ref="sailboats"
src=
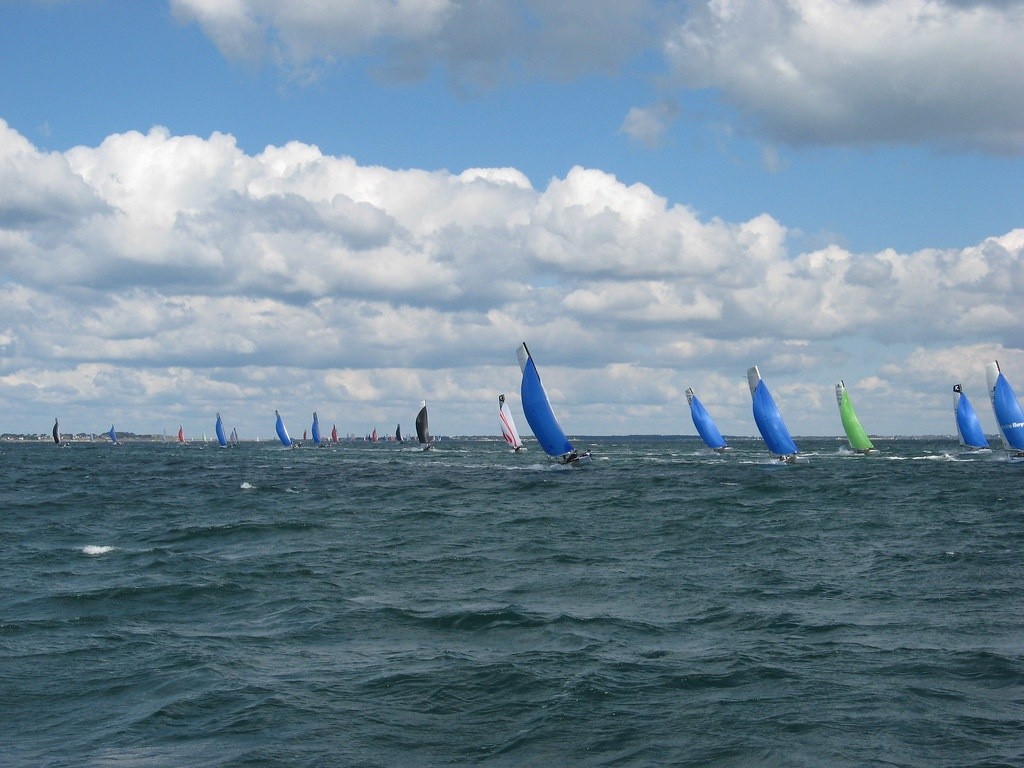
[685,388,735,453]
[108,424,125,446]
[52,417,69,447]
[985,360,1024,459]
[276,409,302,451]
[215,413,233,449]
[498,394,528,453]
[952,383,992,455]
[515,342,592,471]
[364,424,412,444]
[415,400,431,450]
[835,379,881,457]
[747,365,810,463]
[332,424,357,444]
[312,411,331,449]
